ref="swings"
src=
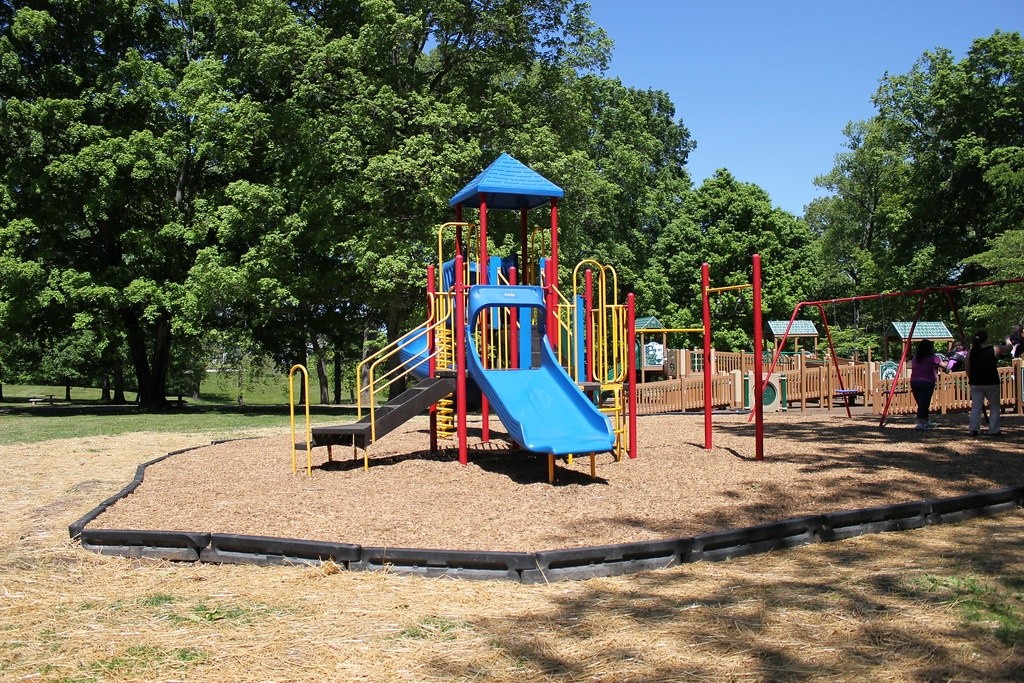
[944,286,1003,373]
[833,299,864,395]
[880,294,910,395]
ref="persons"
[910,339,947,430]
[945,325,1024,372]
[965,331,1013,437]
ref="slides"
[393,325,432,380]
[465,320,616,460]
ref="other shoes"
[970,430,978,436]
[924,420,937,428]
[990,433,1003,436]
[916,424,932,432]
